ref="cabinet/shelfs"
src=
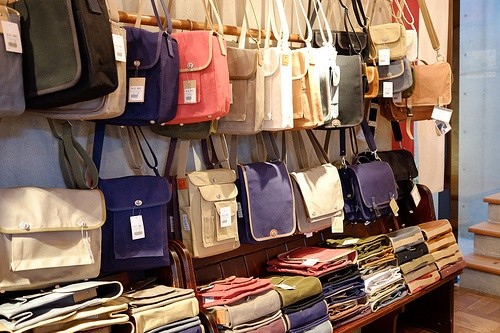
[166,181,469,333]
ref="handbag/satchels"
[335,121,399,226]
[153,0,455,128]
[232,132,297,245]
[0,120,107,292]
[26,0,127,123]
[0,218,468,333]
[350,116,420,205]
[0,0,26,117]
[91,124,173,277]
[175,133,241,259]
[85,0,180,127]
[6,0,119,109]
[285,129,347,237]
